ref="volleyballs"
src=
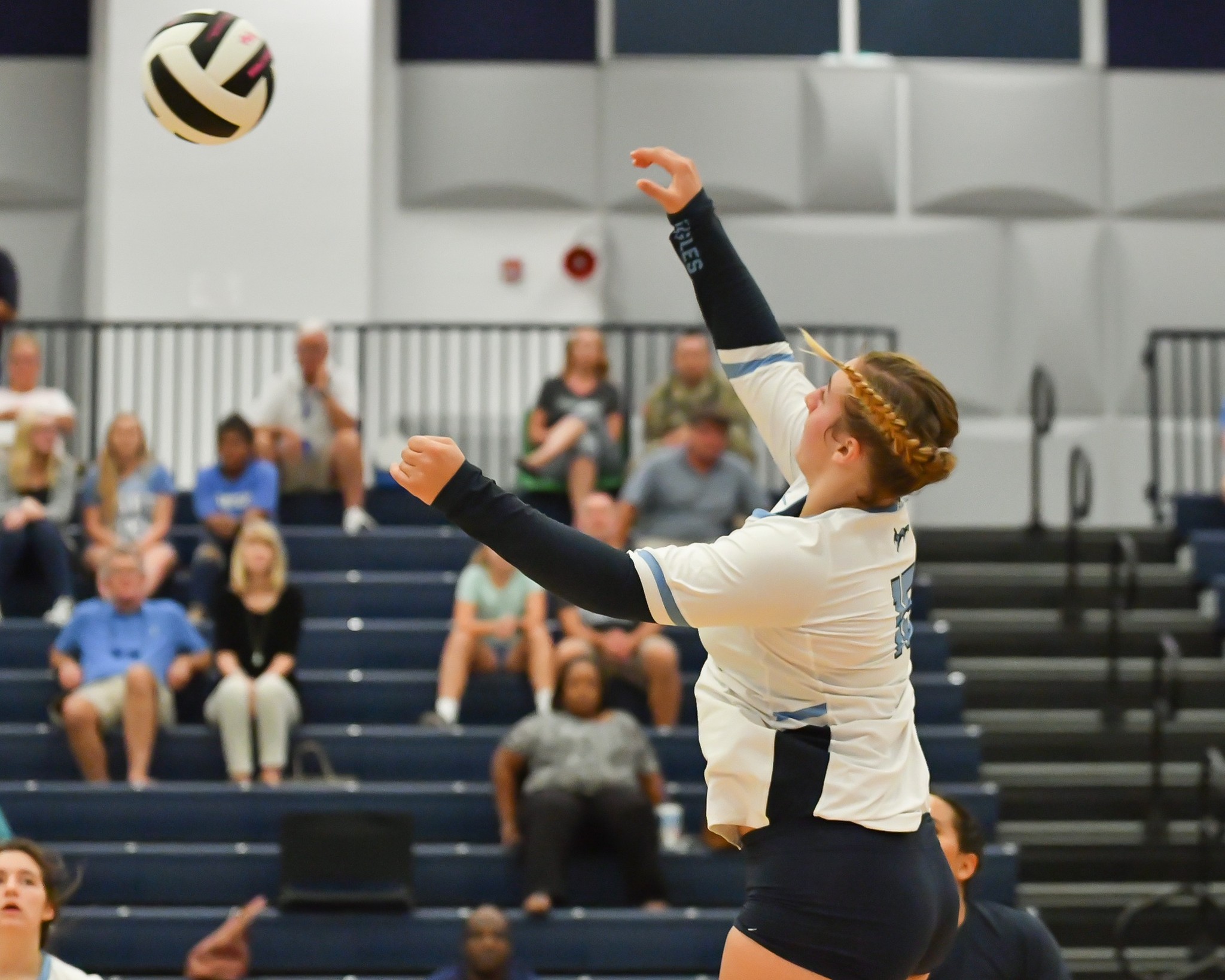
[139,10,275,143]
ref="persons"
[416,324,766,729]
[242,320,379,539]
[0,840,269,980]
[389,143,960,980]
[0,338,304,788]
[924,789,1065,980]
[485,659,674,917]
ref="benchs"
[0,490,1027,980]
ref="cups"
[657,804,685,848]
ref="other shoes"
[418,709,464,735]
[42,598,73,628]
